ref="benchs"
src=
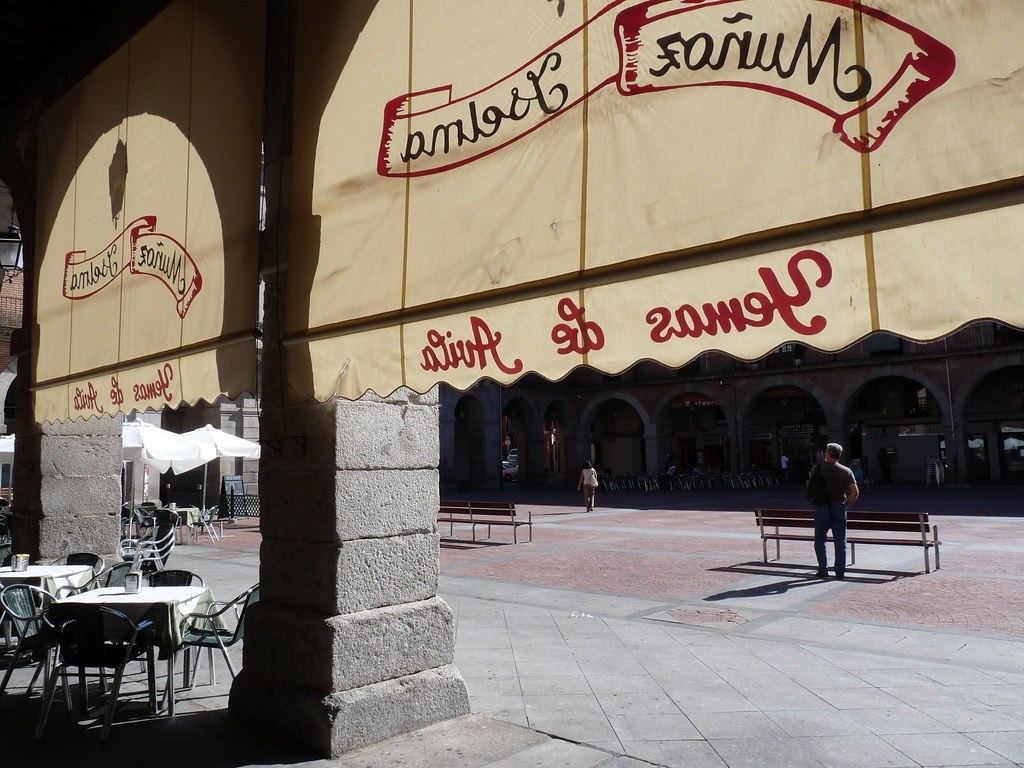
[754,507,941,574]
[438,500,533,545]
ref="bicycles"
[596,469,780,492]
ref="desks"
[32,586,229,719]
[154,508,199,545]
[0,565,100,649]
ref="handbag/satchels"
[591,469,598,487]
[807,464,827,506]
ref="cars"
[507,449,518,463]
[502,461,518,482]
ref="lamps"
[0,202,24,284]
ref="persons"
[664,465,676,491]
[806,443,864,581]
[577,461,599,513]
[781,452,790,484]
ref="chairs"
[597,469,780,492]
[0,501,259,742]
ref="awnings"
[0,0,1023,428]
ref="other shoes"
[836,573,844,579]
[591,509,593,511]
[816,571,828,576]
[587,509,590,512]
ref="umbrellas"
[121,419,261,521]
